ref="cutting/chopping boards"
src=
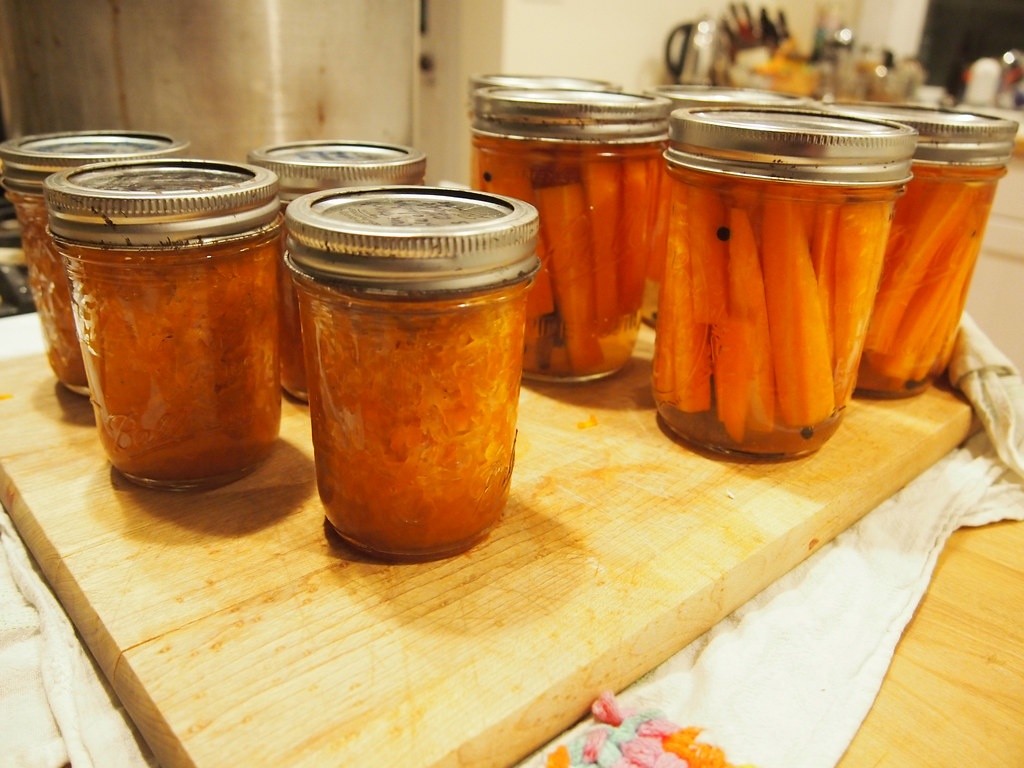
[1,311,984,768]
